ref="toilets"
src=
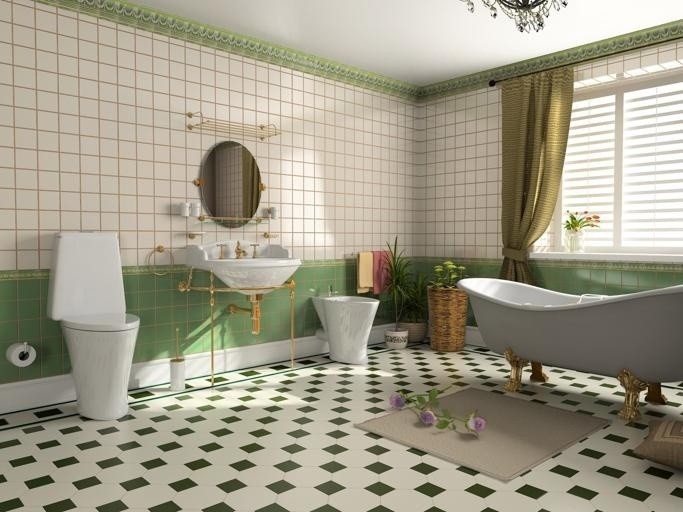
[46,232,142,422]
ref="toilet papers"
[5,342,37,367]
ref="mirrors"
[192,140,267,229]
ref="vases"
[569,230,583,253]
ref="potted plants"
[376,234,469,353]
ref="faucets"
[328,284,338,297]
[235,239,248,258]
[309,286,319,296]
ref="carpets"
[352,385,613,483]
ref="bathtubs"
[454,278,682,385]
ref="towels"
[354,250,391,295]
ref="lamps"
[459,0,569,35]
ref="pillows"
[633,418,683,469]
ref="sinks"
[312,294,381,363]
[209,254,303,294]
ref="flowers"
[560,208,601,235]
[386,385,486,439]
